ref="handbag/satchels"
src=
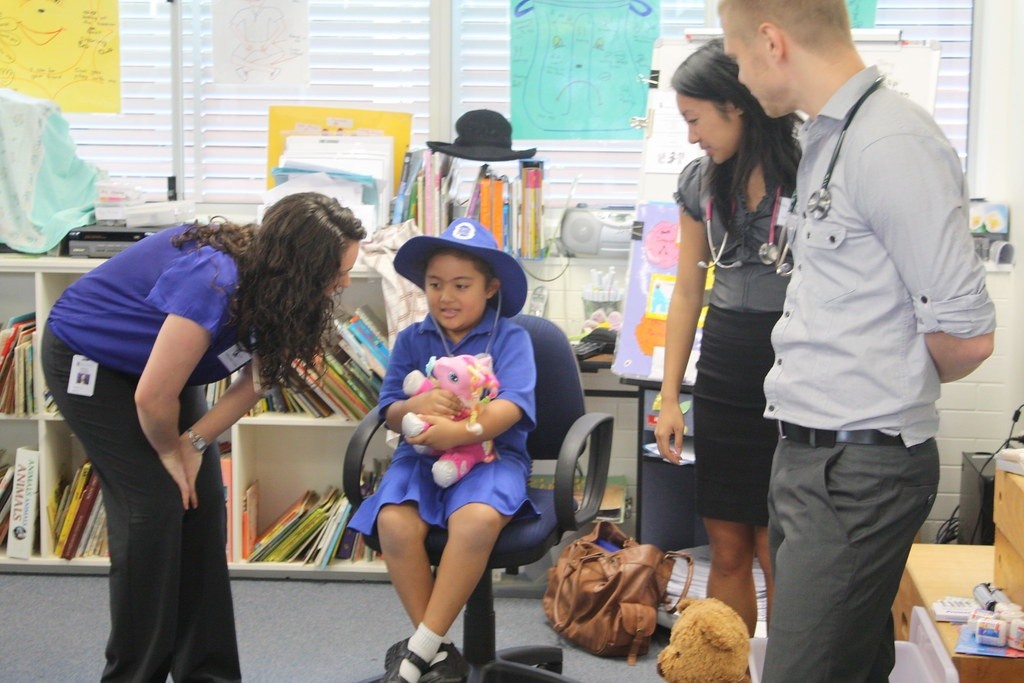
[544,521,693,665]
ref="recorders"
[560,203,635,259]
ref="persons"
[346,217,541,683]
[41,192,367,683]
[717,0,996,683]
[652,39,807,638]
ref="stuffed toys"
[654,597,752,683]
[401,352,500,487]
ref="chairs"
[340,315,616,683]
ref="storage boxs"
[889,466,1024,682]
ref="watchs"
[185,428,207,454]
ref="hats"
[426,110,535,160]
[394,218,526,317]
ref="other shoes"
[418,642,467,683]
[380,637,430,683]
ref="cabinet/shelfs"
[0,255,437,584]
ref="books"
[393,146,546,261]
[0,309,43,563]
[43,362,234,566]
[241,305,390,573]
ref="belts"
[776,419,903,448]
